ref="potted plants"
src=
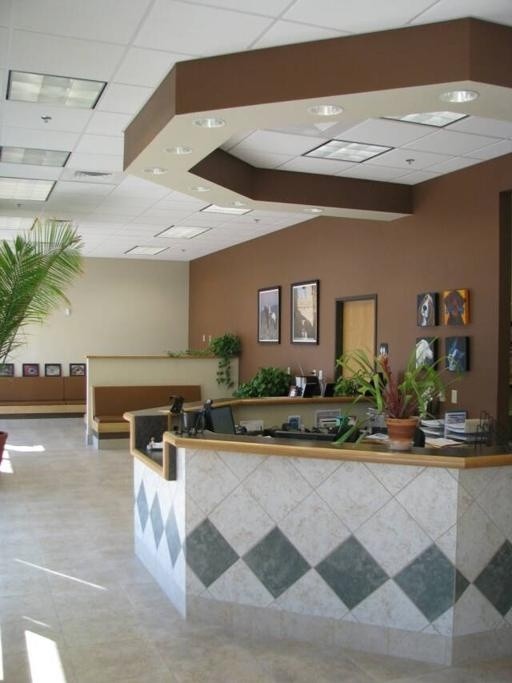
[0,216,86,462]
[332,337,461,452]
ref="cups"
[288,422,298,432]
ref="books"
[417,412,491,450]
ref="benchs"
[91,384,203,450]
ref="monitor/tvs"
[210,406,235,435]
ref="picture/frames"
[257,280,320,343]
[0,363,86,377]
